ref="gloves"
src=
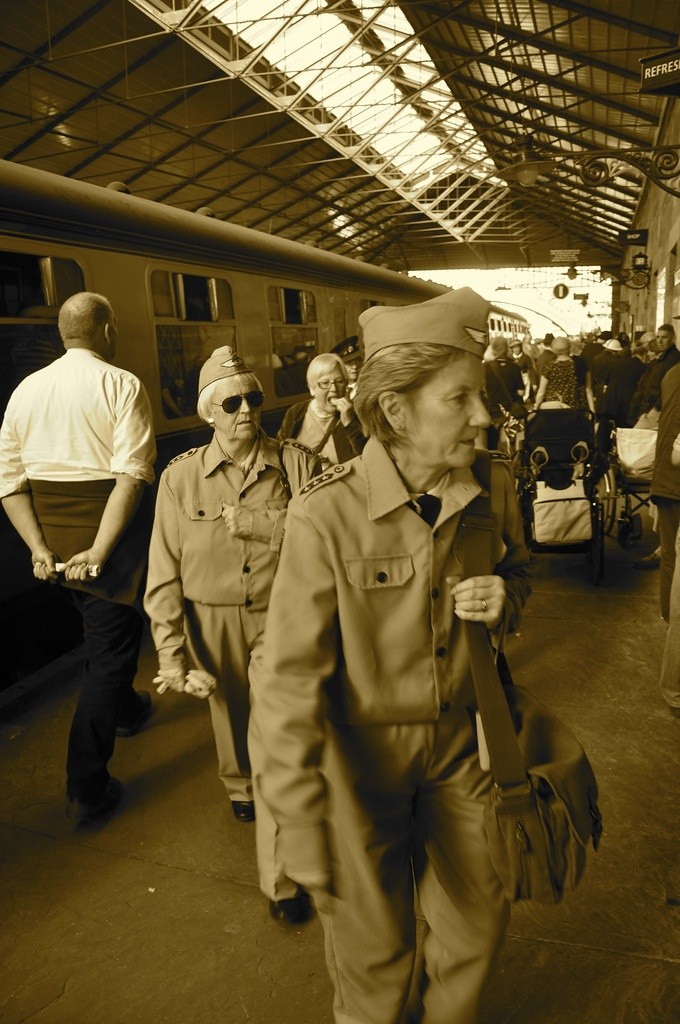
[152,670,218,699]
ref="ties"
[417,494,441,526]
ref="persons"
[475,324,680,450]
[143,345,367,930]
[634,364,680,717]
[0,292,157,822]
[275,335,372,472]
[247,289,531,1024]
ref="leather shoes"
[233,799,253,822]
[631,553,660,570]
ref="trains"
[0,158,530,663]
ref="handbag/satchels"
[483,684,602,905]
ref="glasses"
[212,391,264,413]
[317,379,349,389]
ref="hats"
[509,340,521,347]
[358,286,491,365]
[198,345,251,396]
[329,336,362,363]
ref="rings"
[481,600,487,611]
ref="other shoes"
[116,688,152,736]
[78,776,123,823]
[269,895,305,922]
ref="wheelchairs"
[520,407,606,577]
[595,419,673,534]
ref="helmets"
[602,339,624,350]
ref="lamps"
[493,143,680,200]
[560,264,651,289]
